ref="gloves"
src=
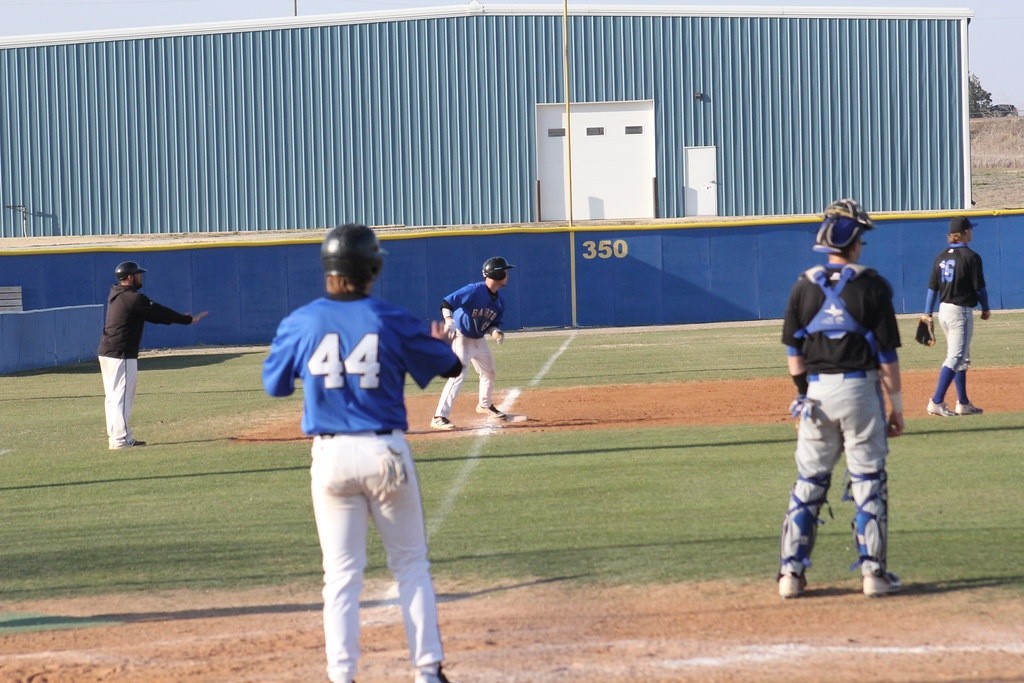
[490,327,504,345]
[443,318,456,340]
[790,396,815,420]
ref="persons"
[777,198,905,601]
[915,216,991,417]
[430,257,516,430]
[96,262,208,450]
[260,224,463,683]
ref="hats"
[949,217,978,234]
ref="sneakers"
[927,398,984,417]
[778,572,805,599]
[476,403,506,420]
[431,417,455,431]
[415,662,448,683]
[862,572,903,598]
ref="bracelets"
[888,392,903,414]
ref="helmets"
[321,223,389,281]
[482,257,515,280]
[114,261,147,281]
[813,199,875,254]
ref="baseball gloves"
[915,317,936,348]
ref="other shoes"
[125,438,147,446]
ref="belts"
[320,429,393,439]
[807,370,867,381]
